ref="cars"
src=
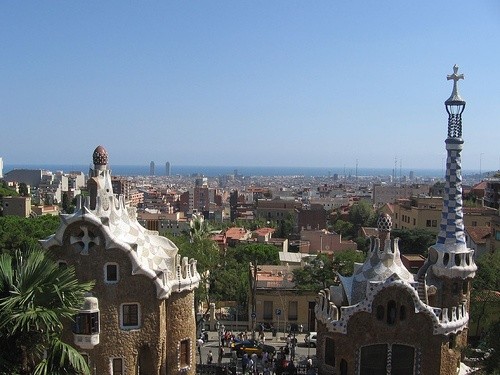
[229,336,276,358]
[304,332,318,347]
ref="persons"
[446,66,464,99]
[196,321,314,375]
